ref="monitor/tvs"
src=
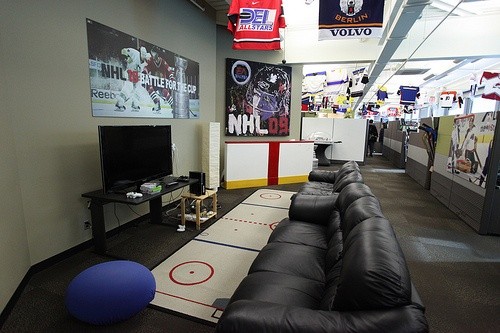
[98,125,173,196]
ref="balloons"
[64,260,156,325]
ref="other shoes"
[369,154,373,158]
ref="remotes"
[166,182,178,186]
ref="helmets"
[140,46,147,61]
[246,67,290,112]
[150,46,160,56]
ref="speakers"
[189,171,206,195]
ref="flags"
[318,0,385,41]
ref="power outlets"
[84,220,90,230]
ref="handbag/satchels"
[369,134,377,143]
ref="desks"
[81,175,199,259]
[180,189,218,231]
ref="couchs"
[216,160,430,332]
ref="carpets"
[145,187,300,326]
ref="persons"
[272,78,290,116]
[449,114,494,186]
[115,45,174,112]
[367,119,387,157]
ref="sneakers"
[131,105,140,112]
[115,103,126,112]
[152,107,161,114]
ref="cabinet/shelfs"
[222,139,315,190]
